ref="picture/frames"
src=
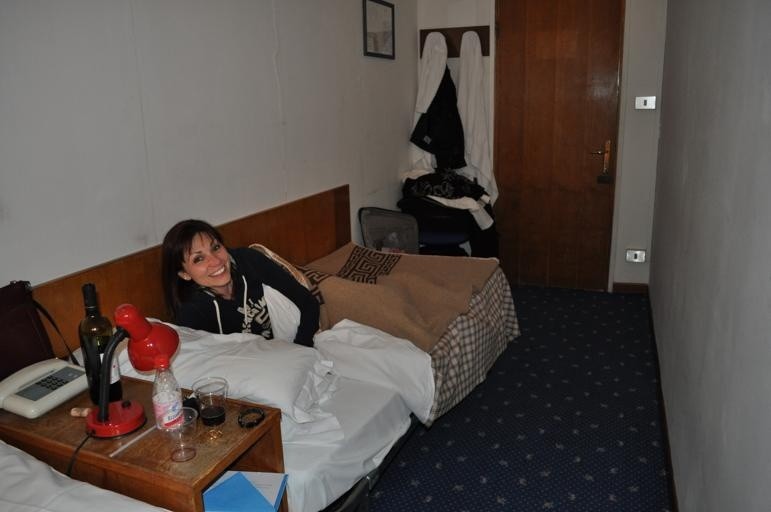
[362,0,395,60]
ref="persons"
[161,219,320,349]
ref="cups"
[157,407,198,463]
[192,376,227,431]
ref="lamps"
[86,303,182,440]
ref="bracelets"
[237,406,264,430]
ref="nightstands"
[0,366,289,512]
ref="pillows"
[70,316,339,440]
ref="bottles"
[151,354,188,441]
[76,284,125,405]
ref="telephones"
[0,357,90,420]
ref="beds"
[32,183,521,511]
[0,435,175,512]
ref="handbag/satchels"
[0,279,80,380]
